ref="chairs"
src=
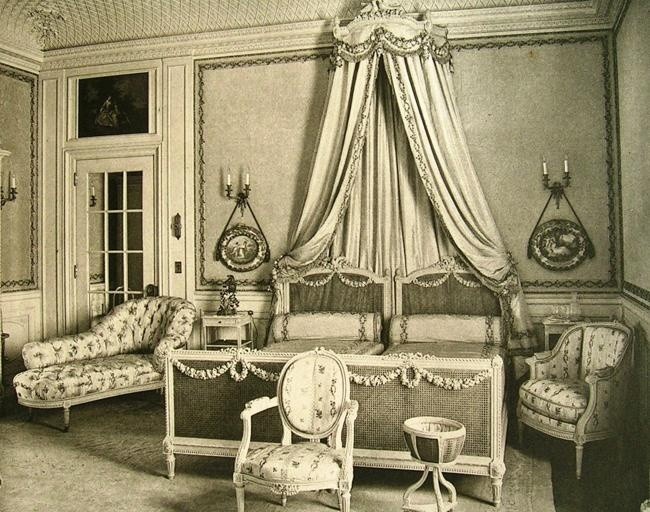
[517,322,635,480]
[232,348,360,512]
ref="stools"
[402,457,458,512]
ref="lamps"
[25,0,66,51]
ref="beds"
[163,254,507,508]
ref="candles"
[245,174,249,185]
[564,160,568,172]
[12,177,16,188]
[228,174,230,185]
[543,163,548,174]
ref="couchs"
[13,296,194,434]
